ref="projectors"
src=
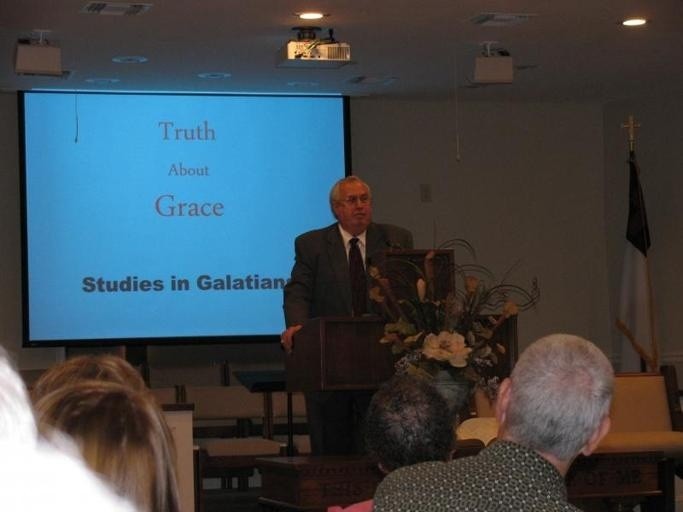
[276,40,351,69]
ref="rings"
[280,339,284,344]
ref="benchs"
[471,373,683,456]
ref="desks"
[157,403,196,512]
[254,452,673,512]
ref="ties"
[349,238,367,315]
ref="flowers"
[364,231,541,402]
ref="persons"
[370,332,616,510]
[279,173,415,354]
[27,352,180,484]
[31,380,185,512]
[325,373,458,511]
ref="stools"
[232,371,302,440]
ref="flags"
[617,146,662,372]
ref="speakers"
[468,53,513,85]
[13,44,62,76]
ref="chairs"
[178,384,290,512]
[269,385,311,511]
[149,385,179,404]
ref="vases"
[431,380,470,415]
